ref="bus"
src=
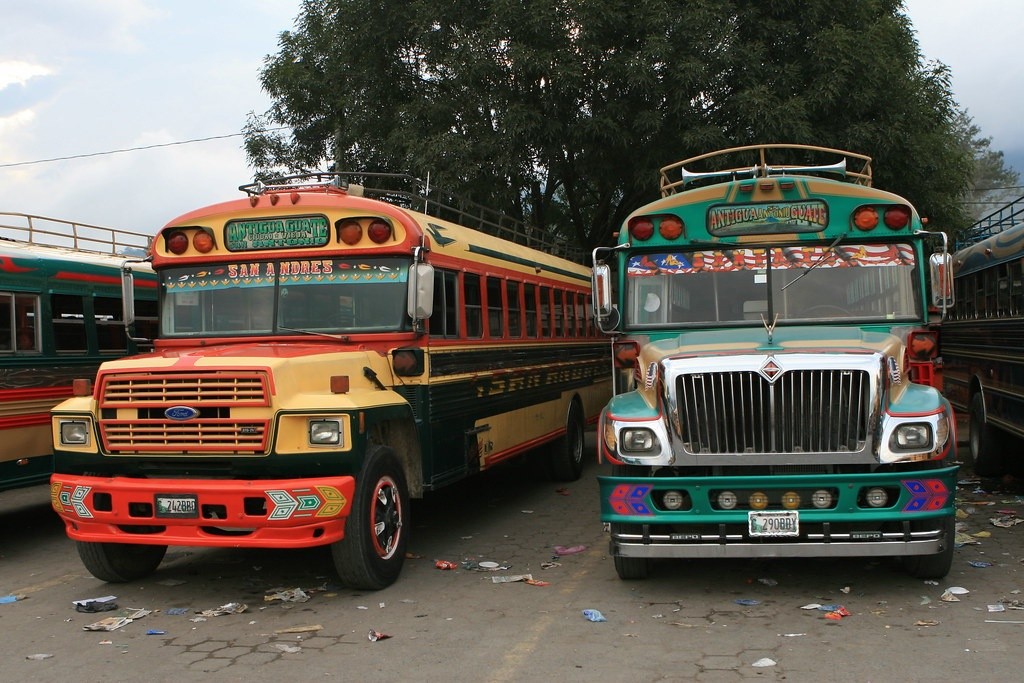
[939,194,1023,478]
[0,213,162,515]
[590,143,961,581]
[49,170,614,593]
